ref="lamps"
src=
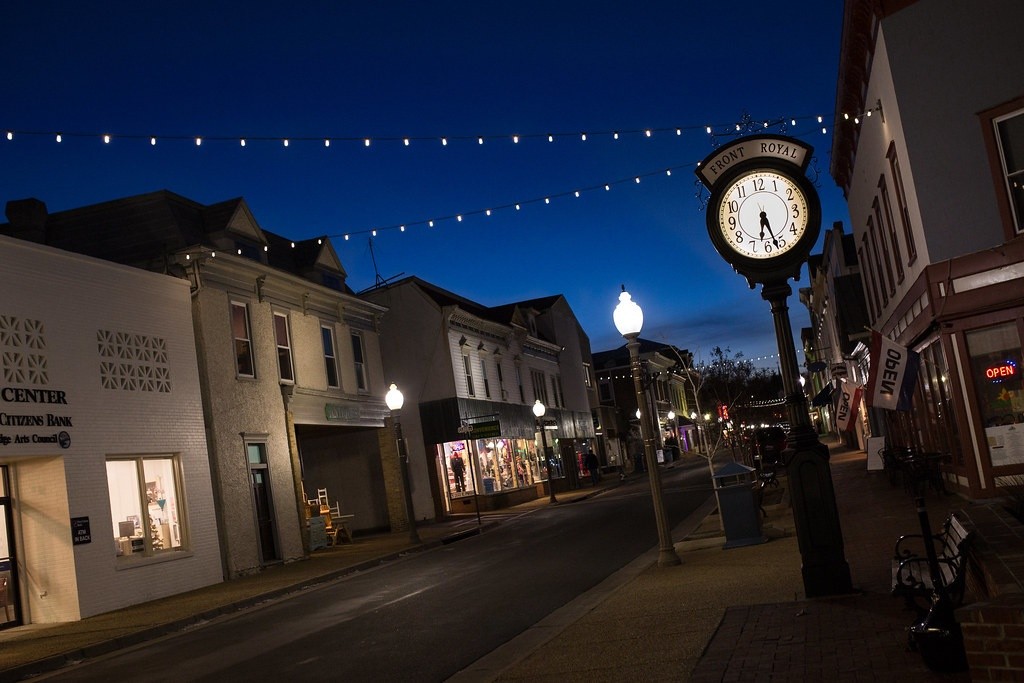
[717,171,806,261]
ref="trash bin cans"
[632,452,646,472]
[759,441,779,473]
[713,462,771,551]
[663,446,680,465]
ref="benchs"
[890,512,975,640]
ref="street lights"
[691,412,703,456]
[704,413,712,449]
[668,410,685,459]
[532,398,557,504]
[612,288,681,572]
[384,381,420,542]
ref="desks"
[332,514,355,543]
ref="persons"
[584,450,600,486]
[451,452,465,492]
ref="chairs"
[320,505,353,549]
